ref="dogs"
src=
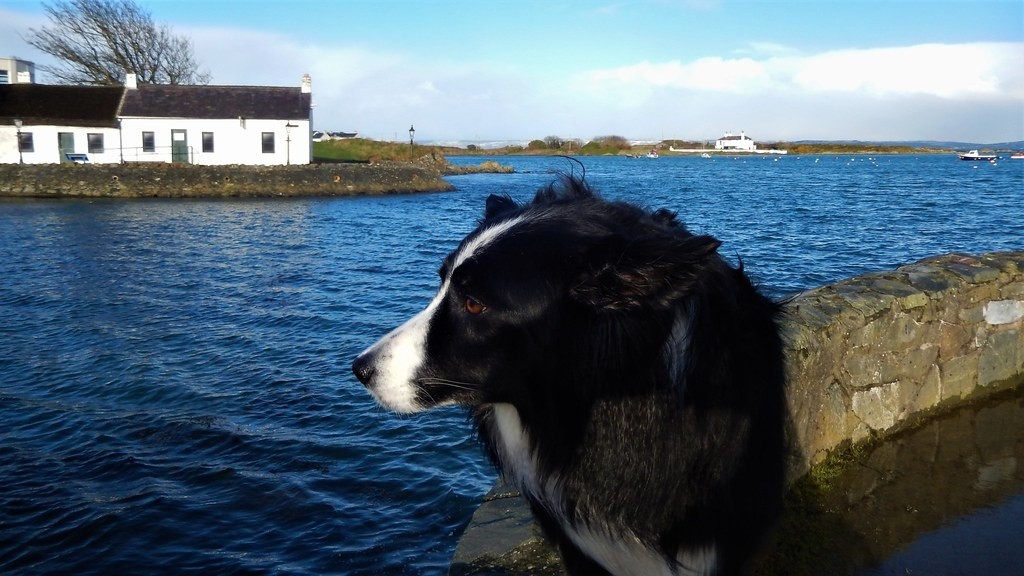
[349,176,793,575]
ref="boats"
[1010,152,1024,158]
[700,152,712,158]
[959,150,998,160]
[646,148,658,158]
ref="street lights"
[409,125,416,163]
[285,122,292,165]
[14,117,23,165]
